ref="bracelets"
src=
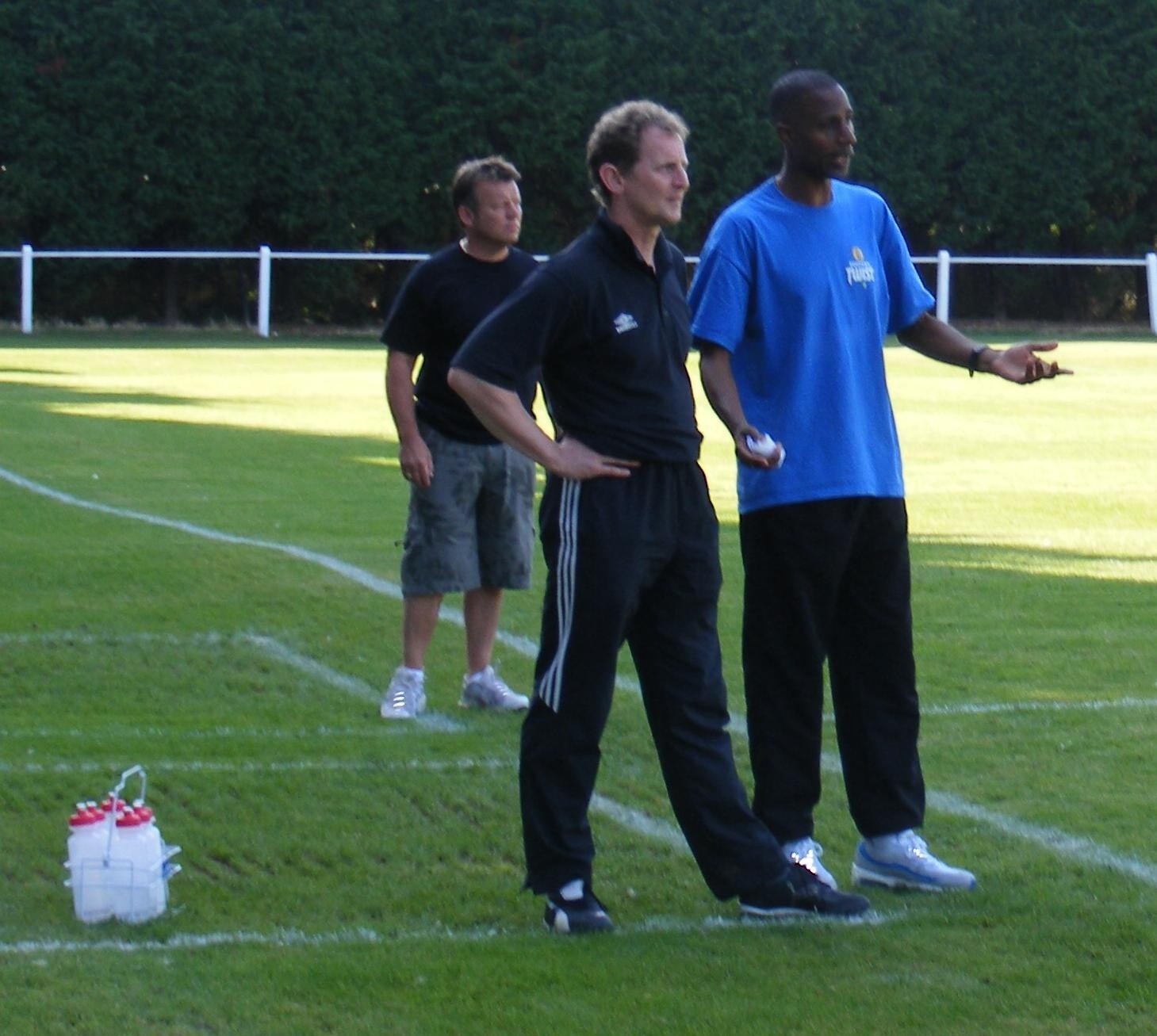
[968,344,990,377]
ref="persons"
[684,70,1073,894]
[380,157,546,718]
[444,98,870,933]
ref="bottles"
[111,806,148,920]
[67,802,115,925]
[86,792,167,914]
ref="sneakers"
[737,864,871,920]
[851,828,978,893]
[379,663,427,719]
[781,835,838,892]
[457,665,530,711]
[543,878,615,935]
